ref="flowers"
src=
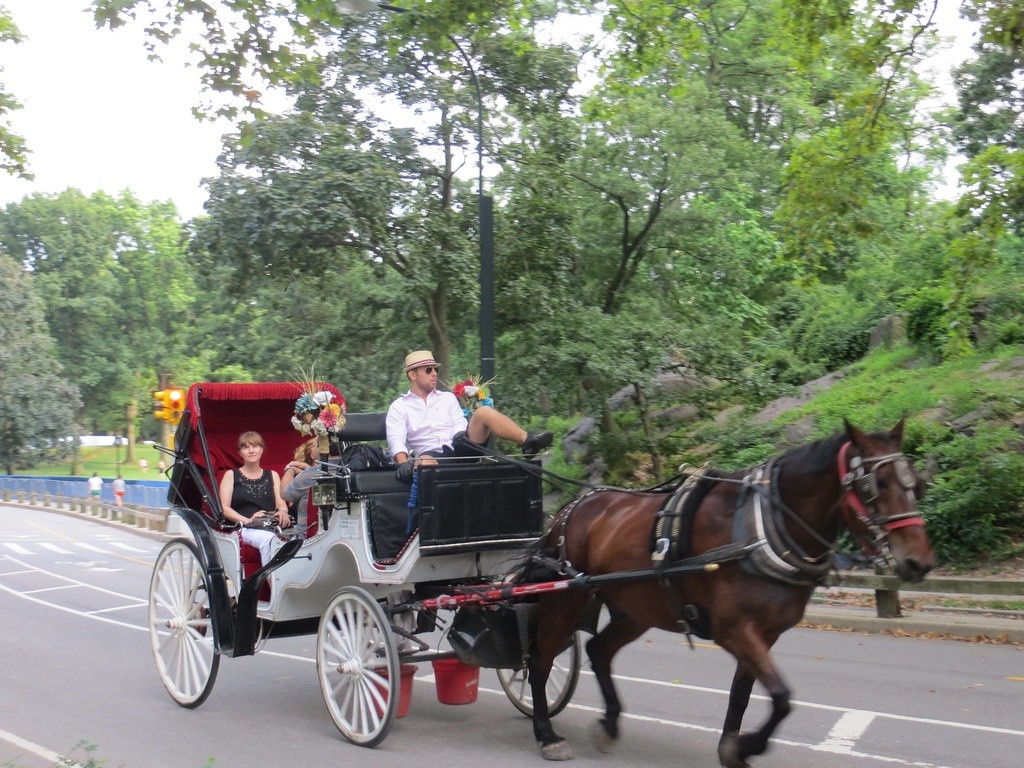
[289,363,348,438]
[441,372,499,418]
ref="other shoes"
[523,431,553,458]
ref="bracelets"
[284,467,297,476]
[279,509,288,514]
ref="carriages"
[148,381,936,768]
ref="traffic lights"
[153,387,185,426]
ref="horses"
[494,415,934,768]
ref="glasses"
[424,366,438,374]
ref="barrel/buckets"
[431,626,481,705]
[367,663,419,718]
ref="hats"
[402,350,441,373]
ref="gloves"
[396,461,413,484]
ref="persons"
[138,457,148,475]
[280,433,347,541]
[219,432,298,590]
[159,459,165,475]
[386,350,553,484]
[88,472,104,501]
[112,474,126,508]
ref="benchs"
[331,408,414,494]
[206,462,318,541]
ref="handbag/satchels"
[339,443,393,470]
[246,509,296,529]
